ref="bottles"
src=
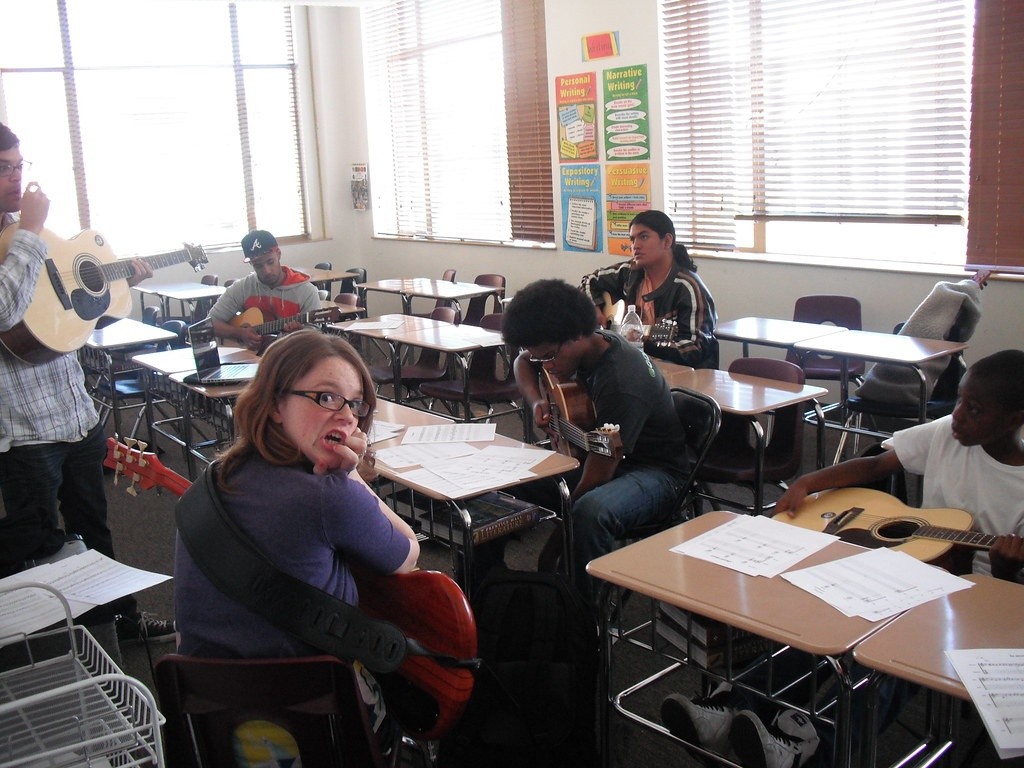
[621,305,645,354]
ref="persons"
[175,326,420,755]
[207,231,324,357]
[578,209,720,370]
[0,123,179,651]
[456,280,692,601]
[659,348,1024,768]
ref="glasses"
[0,160,31,177]
[283,388,371,417]
[530,340,566,366]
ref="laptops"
[188,317,259,384]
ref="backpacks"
[452,568,603,768]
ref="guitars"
[598,290,679,349]
[537,366,623,462]
[102,430,483,745]
[0,220,210,366]
[770,487,998,573]
[215,307,343,356]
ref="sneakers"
[662,691,745,768]
[729,706,821,768]
[115,610,177,646]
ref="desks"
[86,275,1024,768]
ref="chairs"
[91,260,975,768]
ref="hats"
[241,230,278,263]
